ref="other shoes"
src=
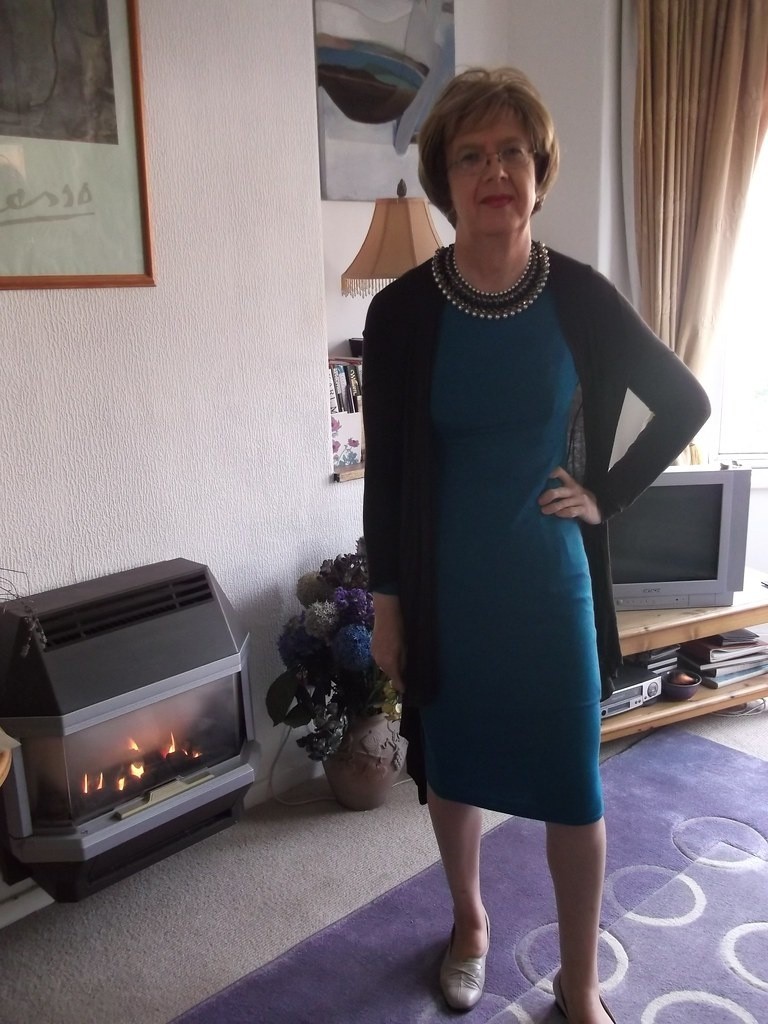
[552,969,616,1024]
[440,910,489,1008]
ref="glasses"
[447,140,537,174]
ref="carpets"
[165,726,768,1024]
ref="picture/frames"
[0,0,158,291]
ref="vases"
[323,711,406,811]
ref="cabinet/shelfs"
[600,582,768,742]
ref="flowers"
[265,535,404,764]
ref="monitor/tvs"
[610,465,751,610]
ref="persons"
[361,68,712,1023]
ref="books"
[624,629,768,688]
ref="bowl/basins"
[660,670,702,701]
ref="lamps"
[340,179,446,300]
